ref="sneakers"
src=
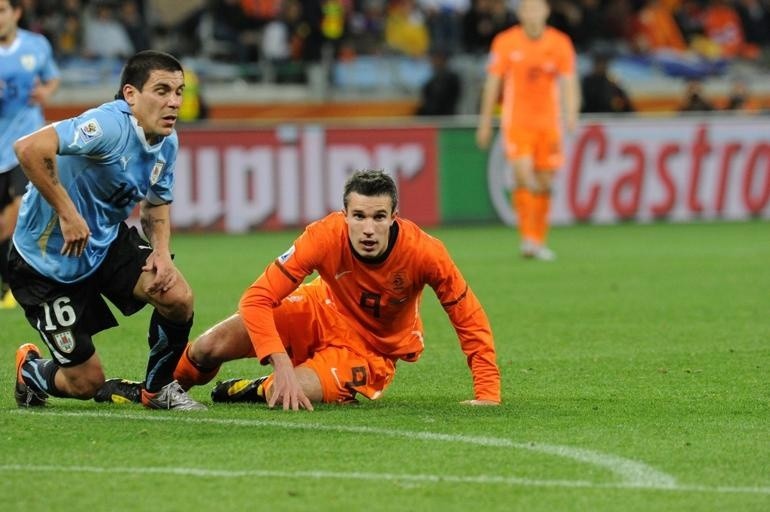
[14,343,49,407]
[95,378,208,413]
[517,240,554,261]
[211,375,269,405]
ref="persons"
[12,49,213,414]
[475,0,582,261]
[578,53,637,114]
[96,169,503,411]
[0,0,58,309]
[413,47,461,116]
